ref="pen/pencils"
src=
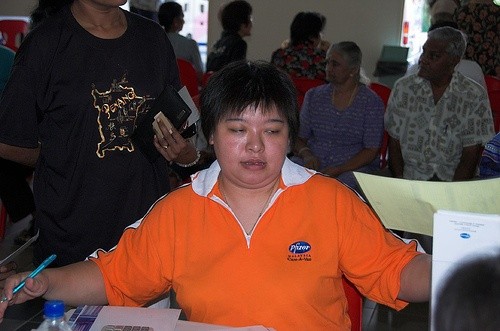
[0,254,57,304]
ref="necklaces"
[219,176,274,235]
[332,82,357,107]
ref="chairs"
[482,74,500,136]
[177,59,215,108]
[369,81,391,167]
[289,75,326,111]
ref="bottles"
[37,300,72,331]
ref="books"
[431,210,500,331]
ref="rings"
[164,144,170,149]
[168,139,173,144]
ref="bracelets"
[176,146,200,167]
[298,147,310,157]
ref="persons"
[205,0,253,73]
[0,61,432,331]
[435,248,500,331]
[474,132,500,179]
[429,0,500,76]
[271,12,496,255]
[0,0,216,269]
